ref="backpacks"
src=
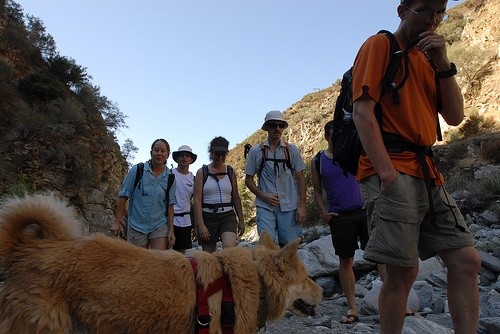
[331,29,406,178]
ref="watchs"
[436,62,458,79]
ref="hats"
[261,110,288,131]
[211,137,228,151]
[172,145,197,164]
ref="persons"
[348,0,482,334]
[110,138,177,252]
[169,145,197,253]
[194,136,244,254]
[310,120,416,323]
[244,112,308,248]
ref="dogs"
[0,190,325,334]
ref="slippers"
[405,306,415,316]
[339,313,358,324]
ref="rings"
[434,38,438,42]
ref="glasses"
[267,122,285,128]
[403,4,449,21]
